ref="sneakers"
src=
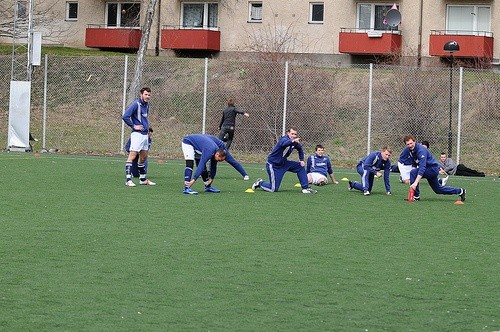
[459,188,466,201]
[399,174,403,183]
[404,195,420,201]
[349,180,353,191]
[252,178,262,191]
[183,188,198,194]
[205,186,220,193]
[302,188,317,194]
[441,176,448,186]
[139,179,156,186]
[364,190,371,196]
[125,180,136,187]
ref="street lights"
[442,39,460,159]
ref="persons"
[251,126,313,194]
[206,135,250,181]
[437,152,486,177]
[181,133,226,194]
[397,133,465,202]
[397,140,429,185]
[348,147,392,196]
[121,87,157,186]
[306,145,339,186]
[217,98,250,150]
[125,128,153,177]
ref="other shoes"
[198,174,201,178]
[317,179,324,186]
[244,175,249,181]
[479,172,485,177]
[208,172,211,177]
